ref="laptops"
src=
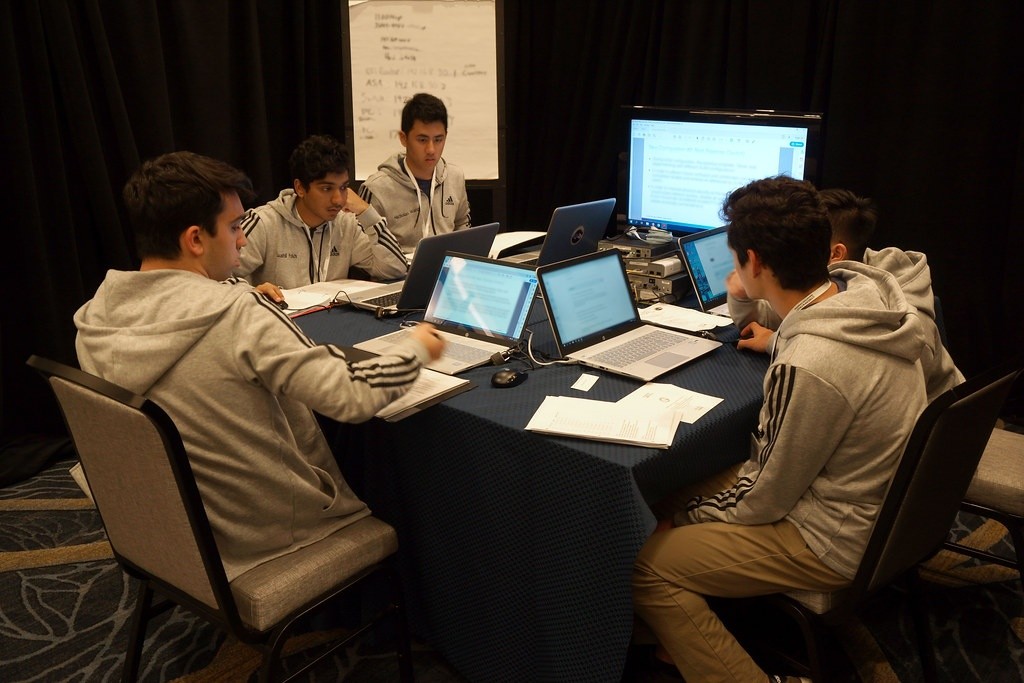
[337,221,501,318]
[679,225,735,320]
[497,198,618,268]
[537,247,723,383]
[353,250,541,377]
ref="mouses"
[263,294,289,312]
[492,368,528,388]
[735,335,755,347]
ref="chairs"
[944,425,1023,572]
[26,354,407,683]
[705,359,1024,683]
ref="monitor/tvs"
[625,104,823,242]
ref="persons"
[723,188,968,411]
[625,172,930,683]
[214,133,410,302]
[72,149,445,586]
[358,92,473,255]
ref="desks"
[291,294,776,683]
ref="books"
[313,340,480,425]
[261,277,395,319]
